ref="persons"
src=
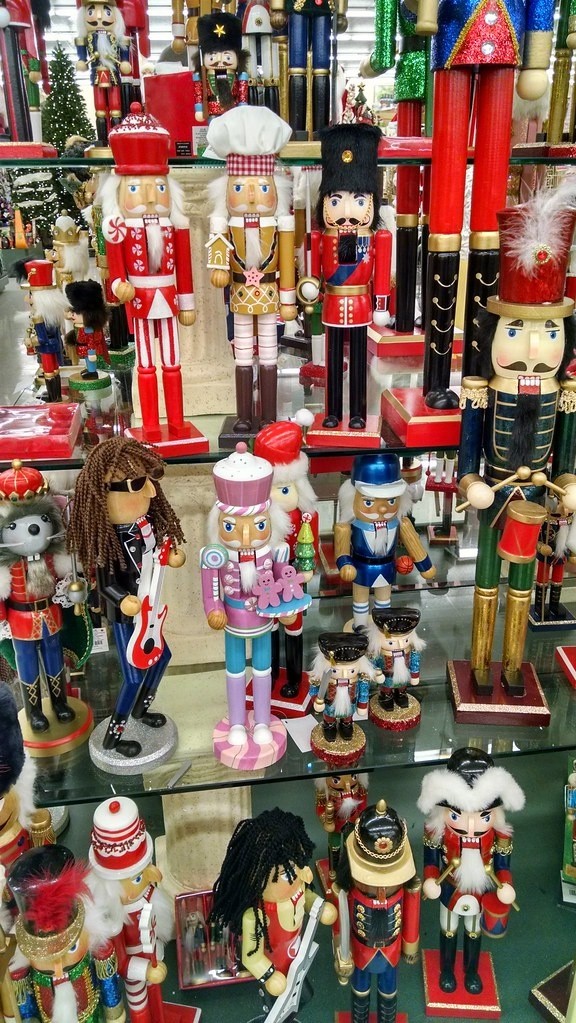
[101,102,196,434]
[296,122,391,429]
[15,0,43,111]
[88,796,173,1022]
[415,0,554,409]
[20,208,111,402]
[357,0,430,333]
[207,106,297,433]
[457,204,576,696]
[255,422,319,699]
[364,607,427,711]
[0,458,83,733]
[418,747,526,994]
[62,437,186,756]
[206,805,338,1023]
[0,681,126,1023]
[200,442,298,745]
[74,0,348,141]
[334,454,436,631]
[187,910,231,973]
[332,800,420,1023]
[304,632,370,742]
[315,769,369,871]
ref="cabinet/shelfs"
[0,157,576,809]
